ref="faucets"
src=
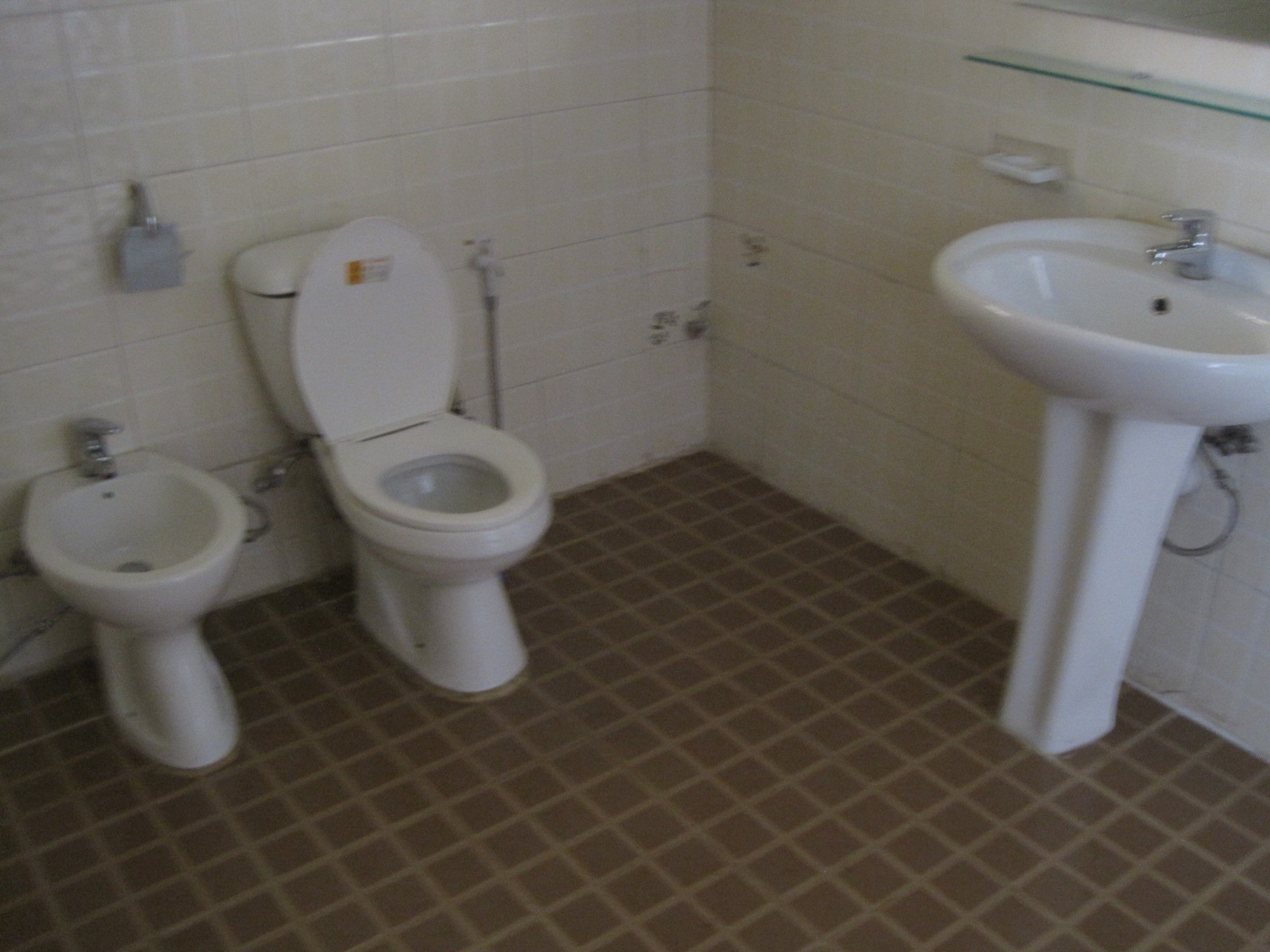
[1145,203,1218,282]
[74,415,123,481]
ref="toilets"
[227,216,554,693]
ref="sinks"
[925,212,1269,430]
[16,447,244,770]
